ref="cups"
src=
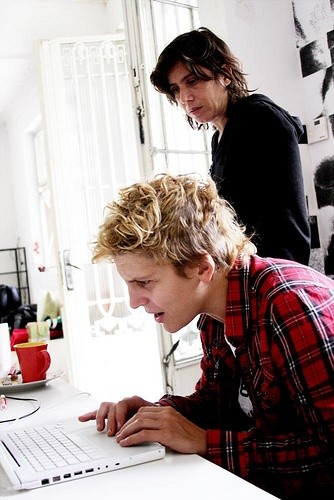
[25,322,39,342]
[13,342,51,382]
[38,322,49,342]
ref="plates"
[0,368,63,394]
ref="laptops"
[0,418,165,492]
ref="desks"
[0,378,281,500]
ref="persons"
[78,174,334,500]
[150,27,311,266]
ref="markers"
[0,394,7,411]
[6,362,18,382]
